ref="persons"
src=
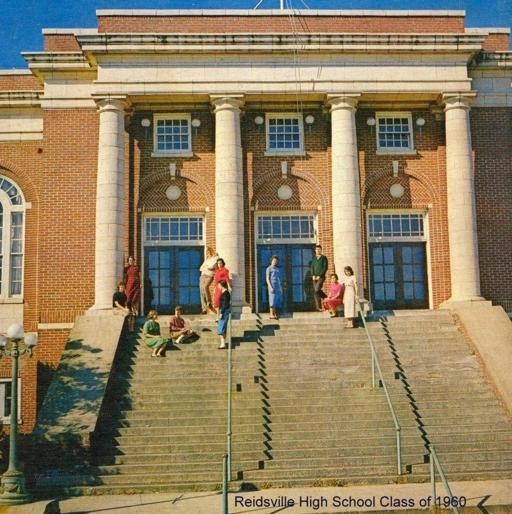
[122,253,144,315]
[322,273,343,317]
[143,309,170,357]
[216,279,231,348]
[266,254,283,318]
[170,305,195,344]
[309,244,328,311]
[342,265,360,328]
[213,257,231,322]
[198,248,221,313]
[113,281,135,333]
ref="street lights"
[1,323,38,504]
